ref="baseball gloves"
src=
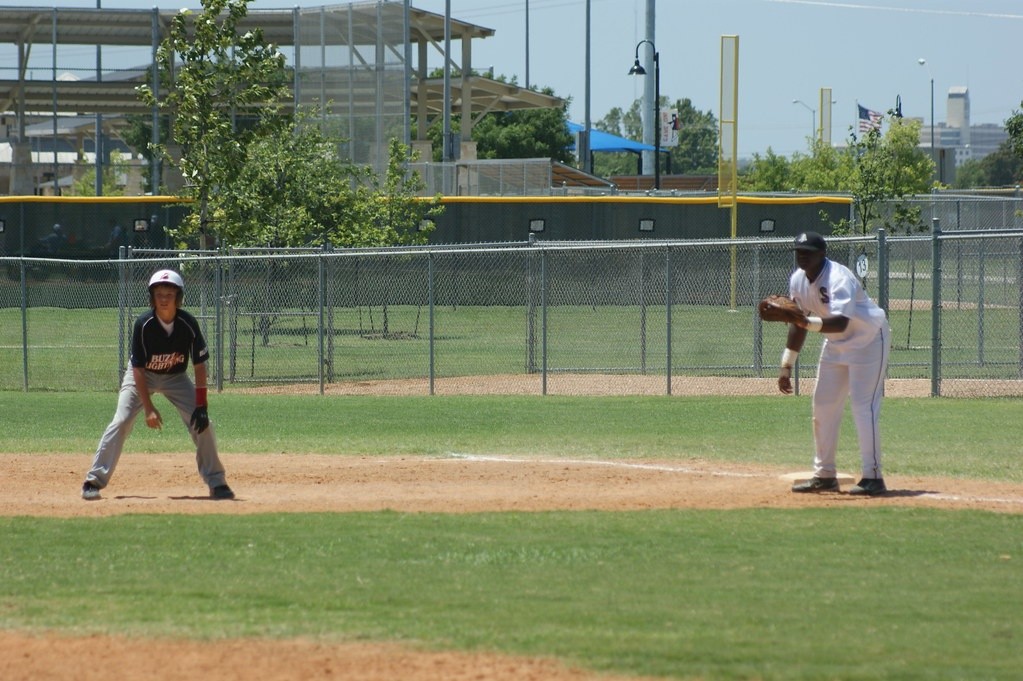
[758,294,805,324]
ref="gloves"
[190,406,209,434]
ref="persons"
[757,230,891,493]
[81,269,234,499]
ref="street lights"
[793,100,837,136]
[917,58,934,161]
[627,40,659,190]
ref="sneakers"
[792,477,839,492]
[82,480,101,500]
[849,479,885,495]
[210,485,235,498]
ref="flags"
[858,104,881,136]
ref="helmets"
[793,231,827,251]
[147,269,183,291]
